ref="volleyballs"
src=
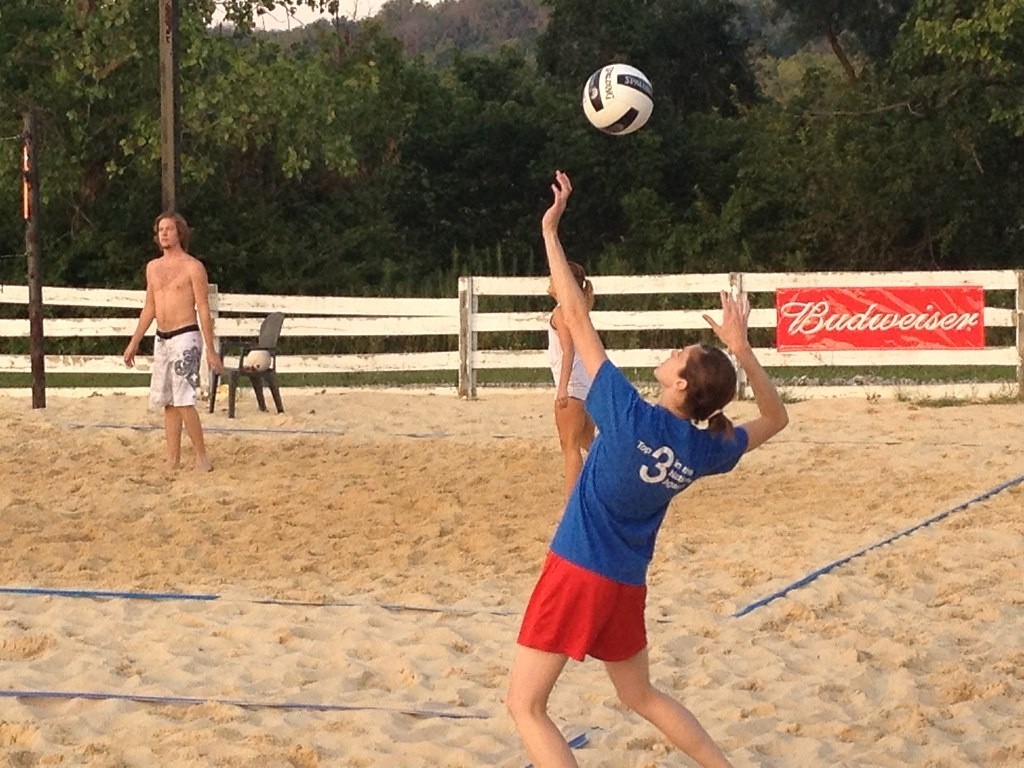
[581,62,656,136]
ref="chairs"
[208,311,285,419]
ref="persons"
[123,211,226,472]
[544,262,607,503]
[498,166,793,768]
